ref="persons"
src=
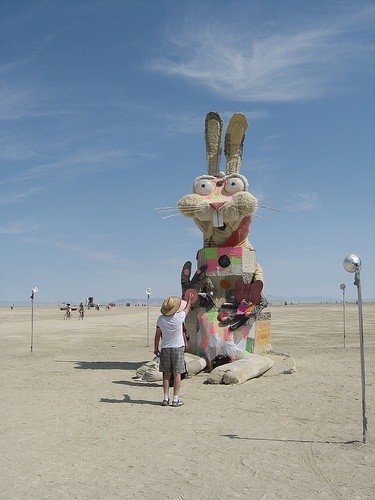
[154,293,194,406]
[66,303,114,320]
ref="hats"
[66,303,71,305]
[160,296,181,317]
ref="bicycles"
[63,311,72,320]
[78,310,84,320]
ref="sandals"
[172,399,184,407]
[162,397,171,406]
